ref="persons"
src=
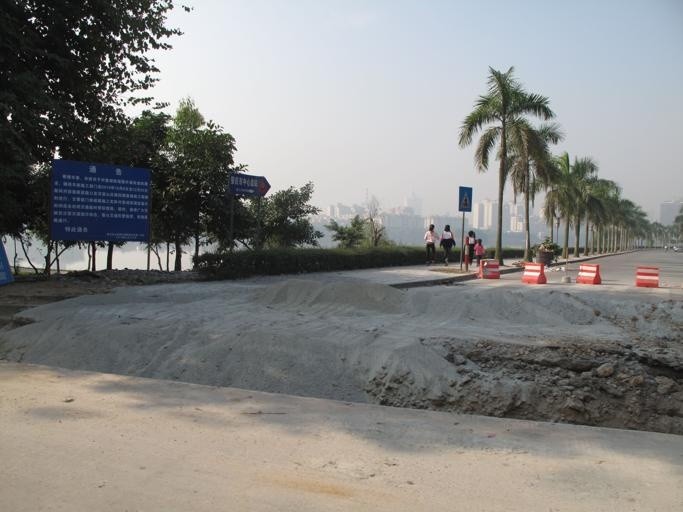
[424,224,439,266]
[440,225,455,265]
[466,231,485,266]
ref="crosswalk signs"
[458,185,472,213]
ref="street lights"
[554,217,560,246]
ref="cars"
[671,243,682,253]
